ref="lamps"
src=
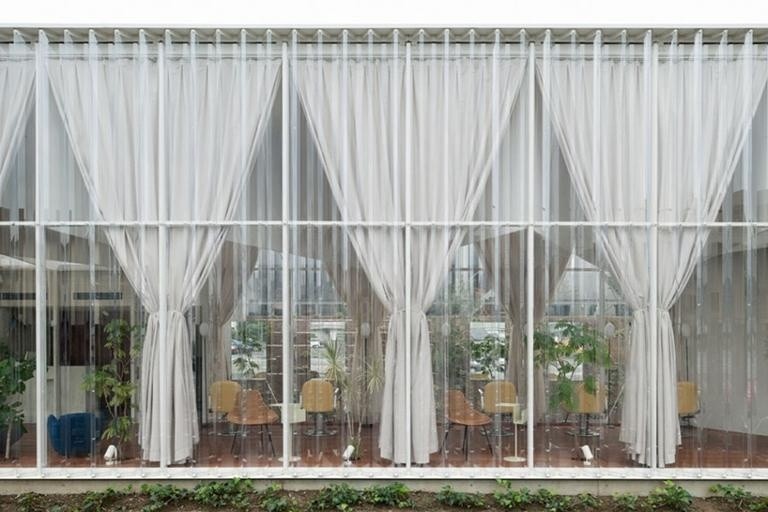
[104,445,117,465]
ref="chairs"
[298,377,340,438]
[48,412,101,458]
[483,380,521,437]
[559,380,603,439]
[676,380,701,435]
[209,379,280,459]
[439,388,495,460]
